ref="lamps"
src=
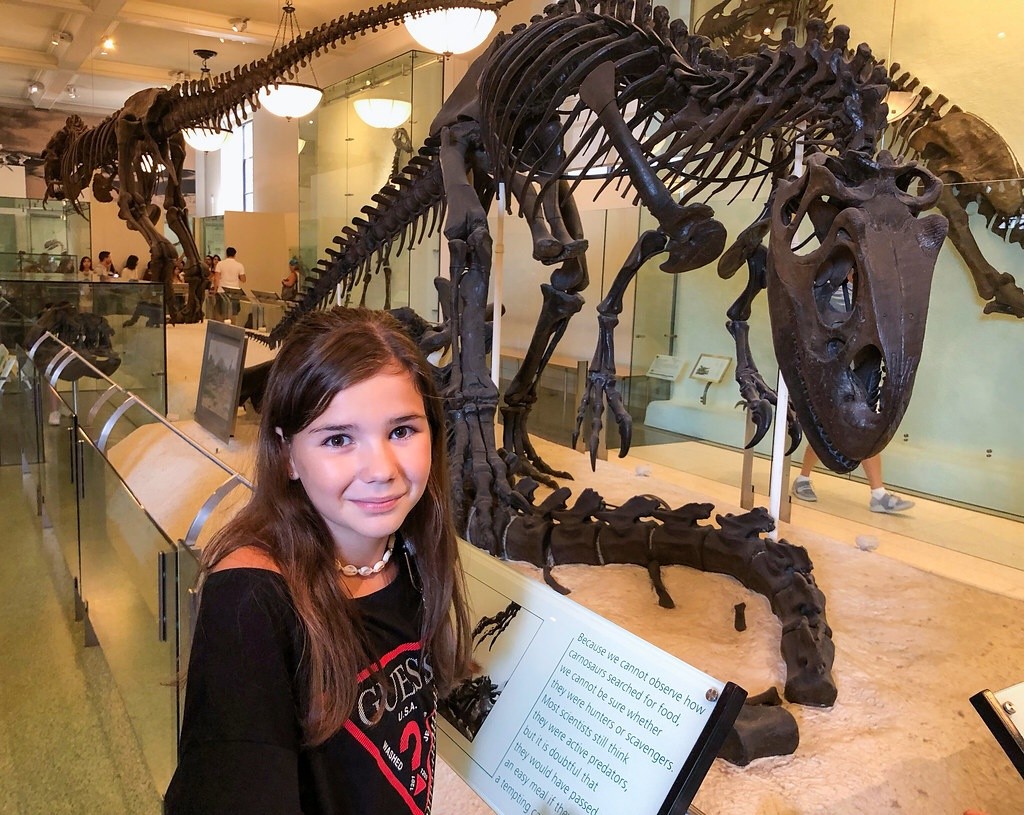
[298,138,306,154]
[403,6,501,59]
[354,98,411,128]
[181,50,234,155]
[68,87,75,99]
[233,18,250,31]
[51,32,73,45]
[256,0,324,122]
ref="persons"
[164,307,499,815]
[173,254,220,293]
[94,251,112,277]
[211,247,247,326]
[121,254,139,282]
[281,254,308,302]
[77,256,99,312]
[44,379,72,425]
[56,251,73,273]
[791,271,915,515]
[142,254,155,282]
[35,253,52,273]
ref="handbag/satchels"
[23,301,121,382]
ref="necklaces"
[331,532,396,577]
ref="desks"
[501,345,650,411]
